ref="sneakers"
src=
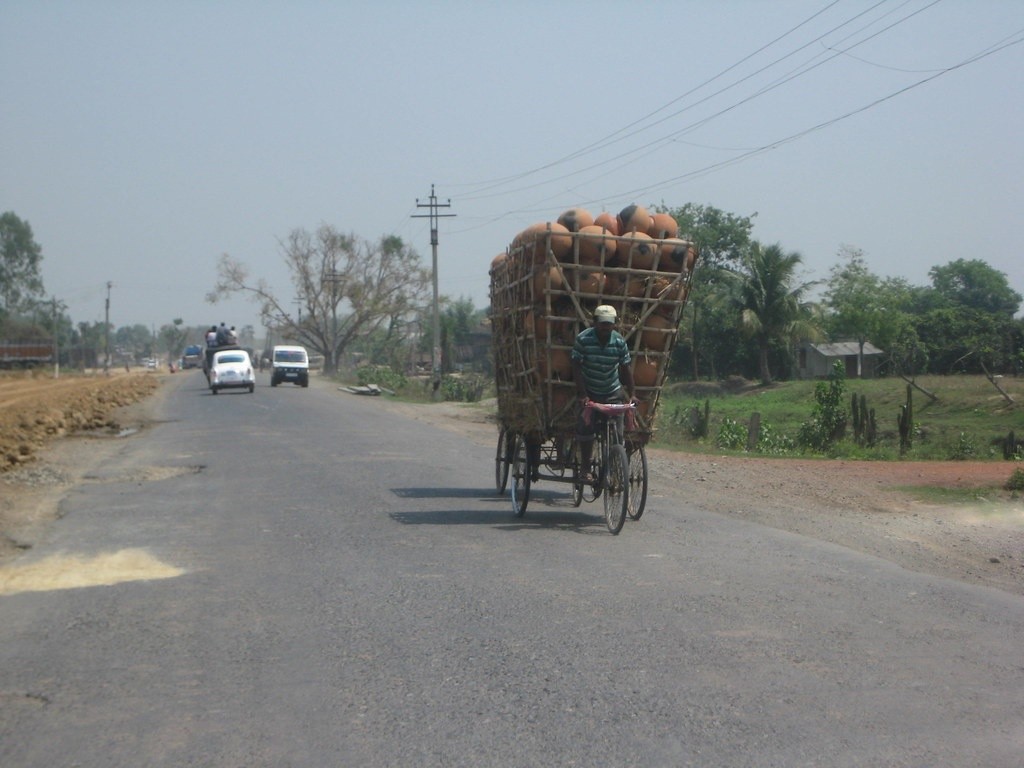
[579,468,592,481]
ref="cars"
[260,351,273,372]
[210,350,256,396]
[147,360,155,369]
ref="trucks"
[0,340,53,372]
[183,346,204,368]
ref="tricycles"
[493,397,650,536]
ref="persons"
[205,321,238,348]
[568,304,640,483]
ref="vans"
[266,345,311,387]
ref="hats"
[594,304,618,324]
